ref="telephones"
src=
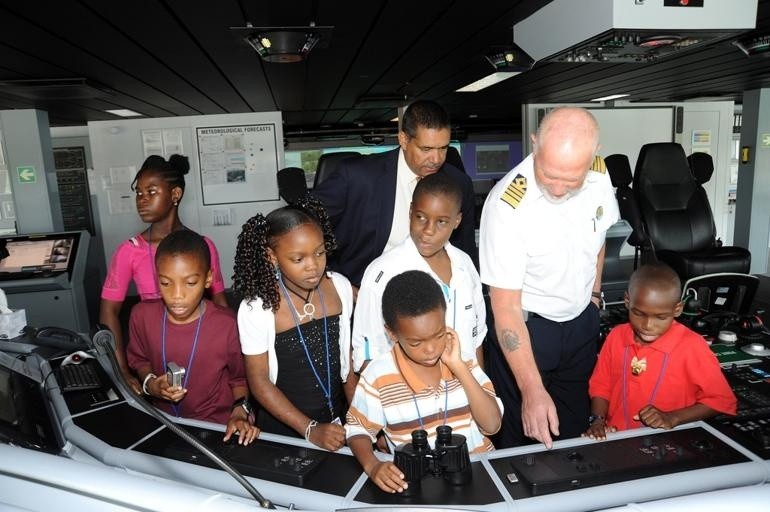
[34,326,90,352]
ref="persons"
[581,260,742,440]
[345,271,503,494]
[98,151,230,397]
[125,227,264,450]
[309,99,479,286]
[352,172,489,383]
[232,194,388,455]
[478,106,623,452]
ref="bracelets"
[302,419,321,444]
[591,289,607,300]
[141,370,156,398]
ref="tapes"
[750,343,764,351]
[718,331,737,342]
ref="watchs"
[232,396,253,420]
[587,412,609,426]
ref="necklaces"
[279,279,320,323]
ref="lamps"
[228,22,333,64]
[480,44,535,72]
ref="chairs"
[681,272,761,316]
[602,142,751,310]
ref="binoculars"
[394,425,473,498]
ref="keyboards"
[60,364,100,391]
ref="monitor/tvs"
[0,232,81,281]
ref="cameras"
[166,361,186,393]
